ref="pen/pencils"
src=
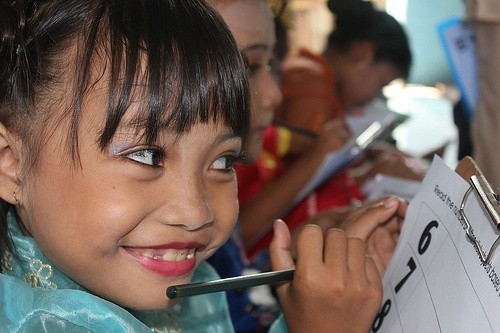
[165,269,296,301]
[270,117,319,140]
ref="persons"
[195,0,360,333]
[224,121,428,265]
[0,0,411,332]
[272,0,442,184]
